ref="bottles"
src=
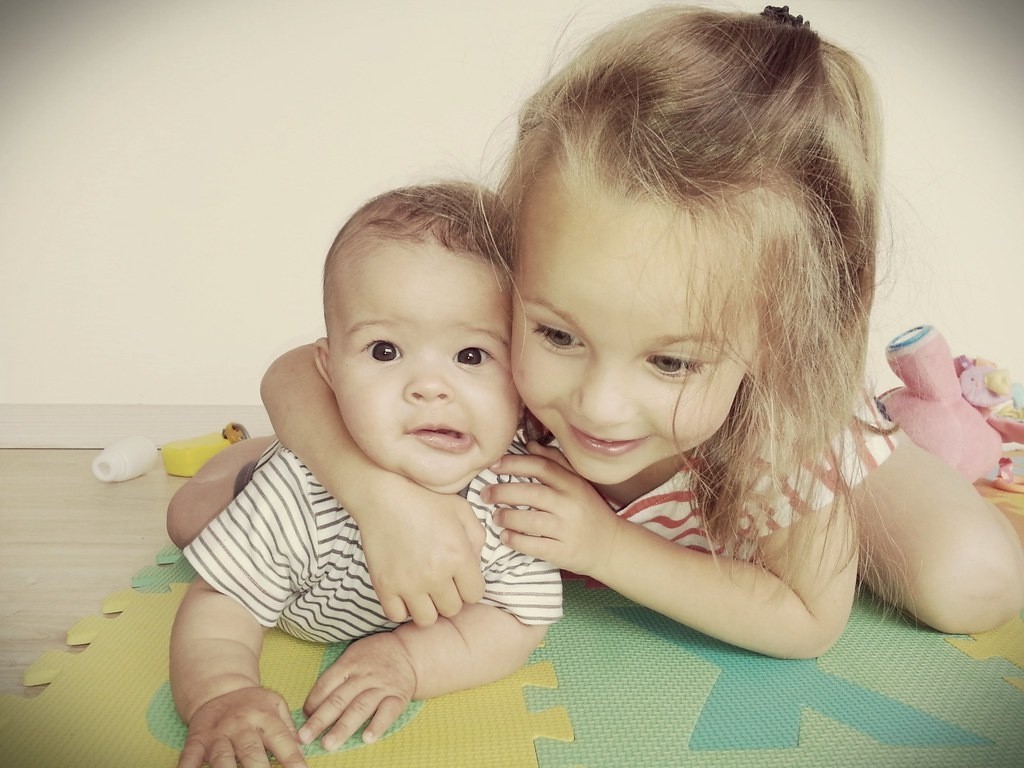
[93,436,158,481]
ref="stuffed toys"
[874,325,1024,490]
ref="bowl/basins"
[162,435,230,476]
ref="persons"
[258,6,1023,662]
[166,184,560,767]
